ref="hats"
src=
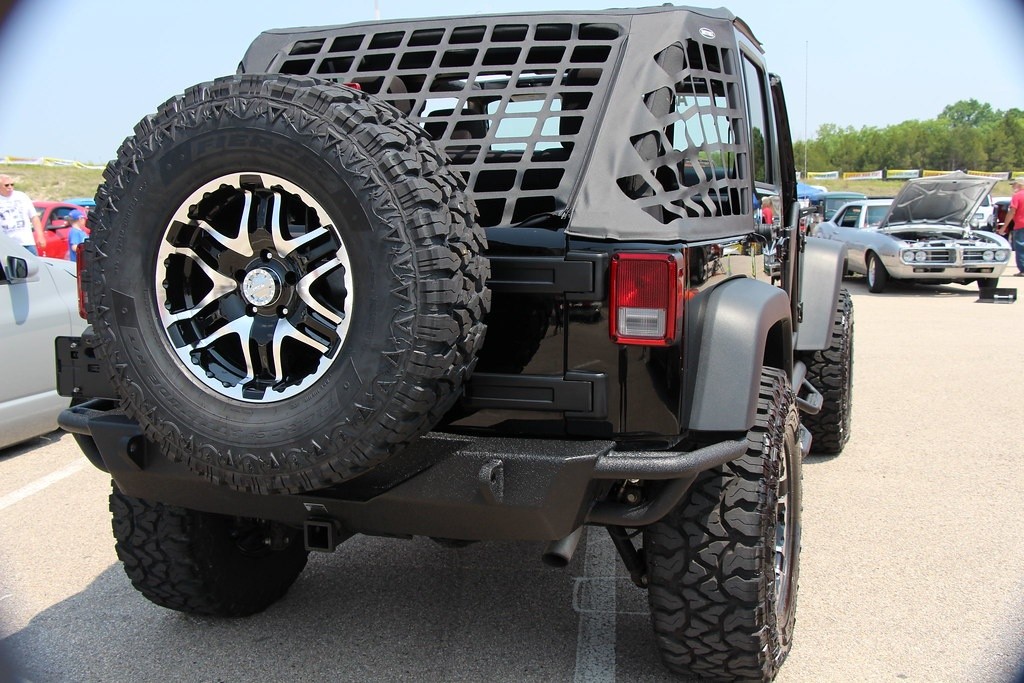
[1009,177,1024,186]
[69,210,84,220]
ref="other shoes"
[1014,272,1024,277]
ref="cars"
[54,3,853,683]
[0,198,97,450]
[753,170,1013,293]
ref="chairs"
[423,109,491,151]
[533,68,685,224]
[41,212,58,219]
[350,76,411,115]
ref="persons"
[999,177,1024,277]
[761,196,773,224]
[0,174,46,256]
[69,210,89,262]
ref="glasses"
[3,184,14,187]
